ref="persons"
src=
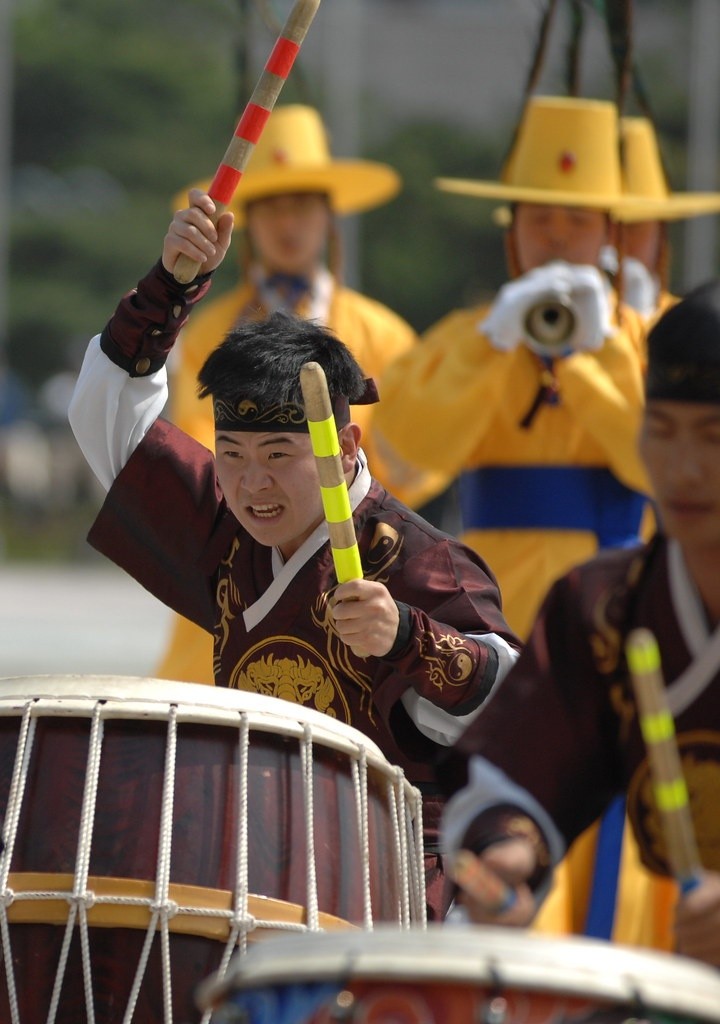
[606,116,719,342]
[354,91,682,954]
[154,102,418,685]
[65,186,532,922]
[436,279,719,971]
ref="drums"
[2,666,419,1024]
[198,922,719,1023]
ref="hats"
[171,100,403,228]
[608,114,718,224]
[435,92,671,211]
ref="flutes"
[525,293,578,345]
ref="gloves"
[560,264,613,352]
[598,244,620,276]
[620,256,658,323]
[480,258,570,352]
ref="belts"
[446,459,652,551]
